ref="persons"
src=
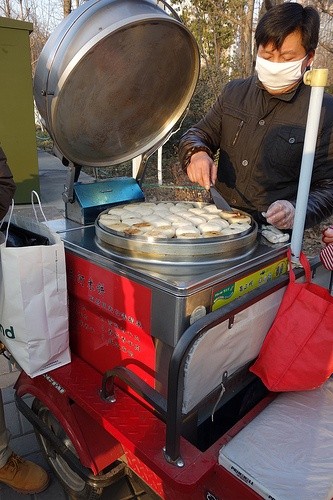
[178,2,333,238]
[0,145,50,494]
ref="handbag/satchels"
[0,191,73,378]
[249,248,333,391]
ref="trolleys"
[1,0,333,500]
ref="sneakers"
[0,451,51,494]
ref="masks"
[255,54,309,90]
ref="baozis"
[99,202,252,240]
